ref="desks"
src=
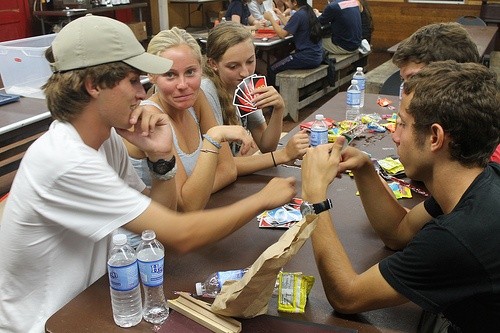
[200,12,333,79]
[387,25,500,61]
[45,92,431,333]
[33,3,148,36]
[0,74,153,196]
[171,0,223,30]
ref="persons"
[357,0,374,45]
[392,21,500,164]
[300,62,500,333]
[119,25,256,213]
[200,21,310,176]
[264,0,324,93]
[225,0,292,26]
[0,13,299,333]
[315,0,364,54]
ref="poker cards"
[329,132,354,158]
[258,196,307,229]
[232,74,268,117]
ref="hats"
[45,11,173,75]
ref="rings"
[246,129,253,136]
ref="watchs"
[300,199,333,219]
[146,155,176,175]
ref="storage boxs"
[126,21,148,41]
[0,34,58,99]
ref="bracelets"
[203,134,222,149]
[200,149,220,154]
[271,151,277,167]
[147,167,177,180]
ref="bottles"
[220,17,226,24]
[353,67,365,108]
[310,114,328,146]
[194,269,284,299]
[291,10,296,18]
[107,234,142,328]
[345,80,360,121]
[399,81,405,107]
[135,230,169,324]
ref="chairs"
[457,16,487,27]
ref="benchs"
[276,45,373,122]
[362,57,400,88]
